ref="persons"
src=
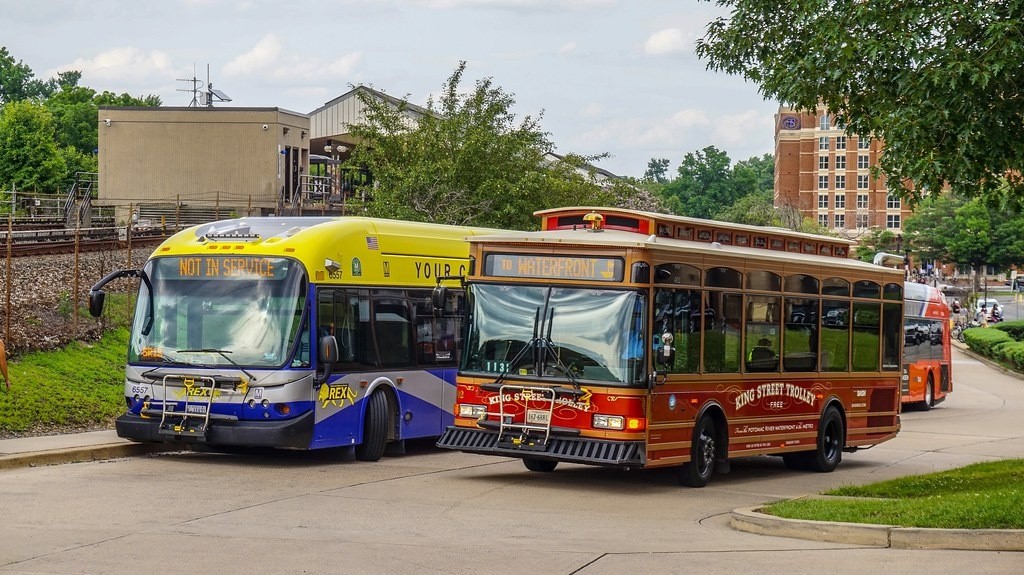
[950,298,987,328]
[991,304,1000,323]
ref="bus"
[89,214,534,461]
[531,199,853,260]
[436,212,906,488]
[902,280,954,410]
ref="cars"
[1012,274,1024,294]
[904,322,942,348]
[973,298,1004,323]
